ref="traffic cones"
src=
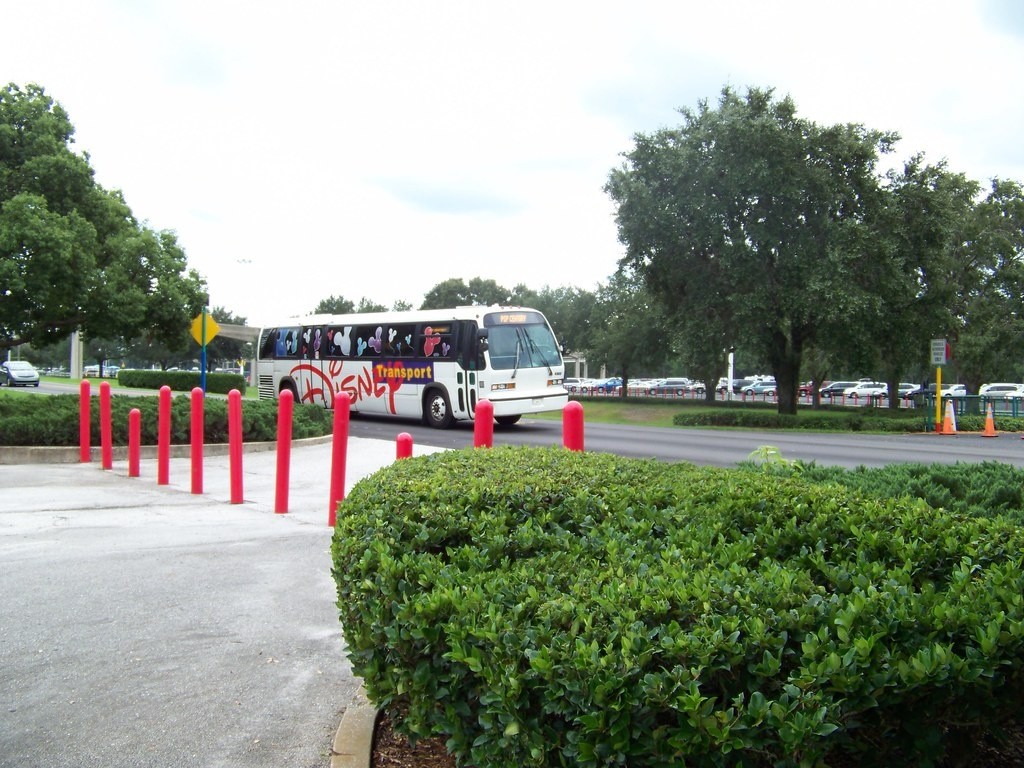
[979,402,1000,437]
[940,401,958,435]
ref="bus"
[258,303,569,429]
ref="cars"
[82,361,252,387]
[0,361,41,388]
[562,375,1024,402]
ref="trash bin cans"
[912,393,928,409]
